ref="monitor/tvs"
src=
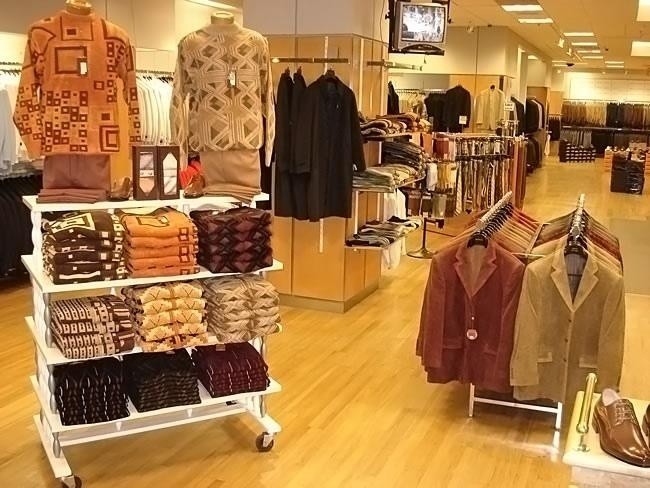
[394,2,448,51]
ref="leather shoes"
[106,178,133,200]
[184,175,204,198]
[593,388,650,467]
[614,145,625,152]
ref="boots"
[631,154,645,162]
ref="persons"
[11,0,141,188]
[168,10,276,185]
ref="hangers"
[282,59,337,85]
[0,61,22,78]
[465,191,589,263]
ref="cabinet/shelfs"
[344,126,426,250]
[20,192,285,488]
